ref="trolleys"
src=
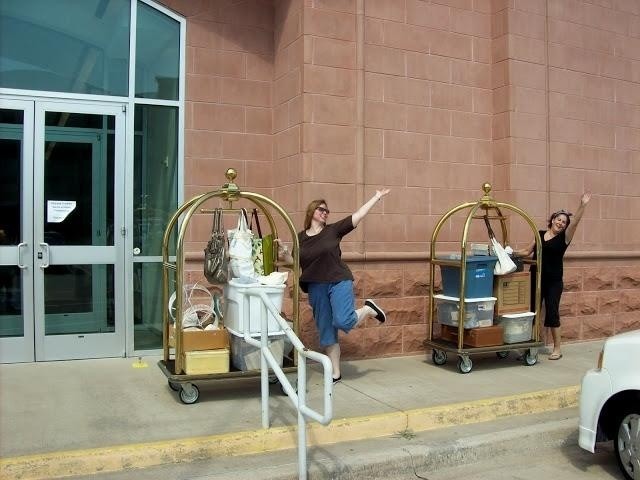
[158,167,300,404]
[109,194,172,326]
[428,183,547,374]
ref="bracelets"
[372,194,381,202]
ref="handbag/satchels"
[227,208,258,285]
[249,208,276,277]
[483,215,517,276]
[203,208,229,285]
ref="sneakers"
[516,349,530,361]
[333,374,342,385]
[364,298,387,325]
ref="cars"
[575,328,640,480]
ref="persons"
[504,190,593,361]
[270,186,395,387]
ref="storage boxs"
[172,277,287,376]
[435,252,537,347]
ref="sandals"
[548,352,563,360]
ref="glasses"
[316,206,329,214]
